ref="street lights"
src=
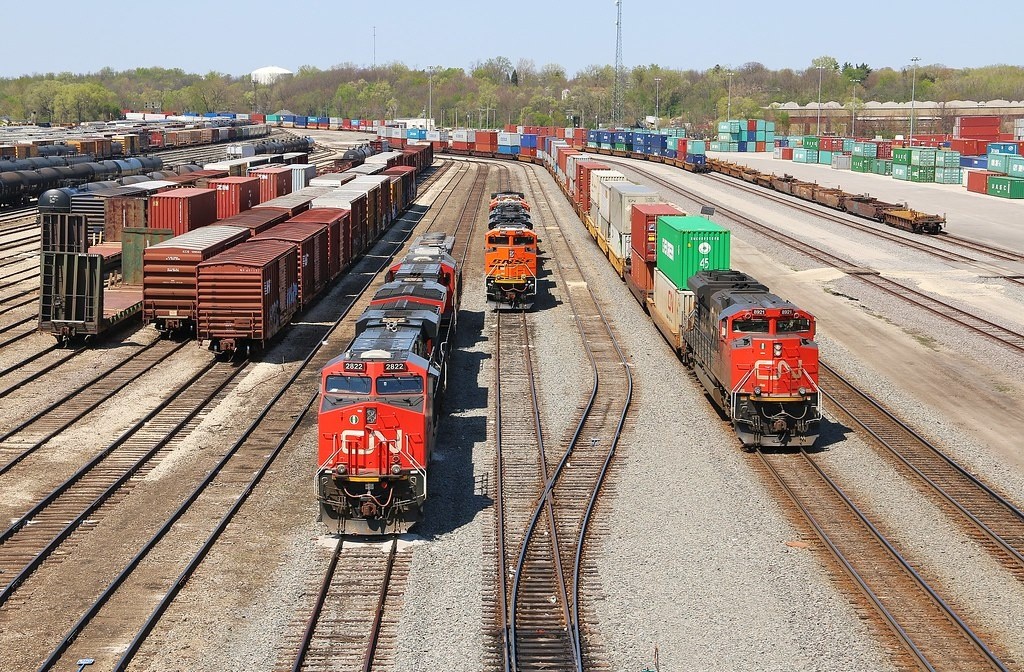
[816,66,826,136]
[654,78,661,117]
[727,72,734,121]
[849,80,860,137]
[908,57,922,146]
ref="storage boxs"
[0,113,1024,338]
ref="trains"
[0,113,948,364]
[485,190,537,310]
[679,270,823,452]
[315,230,462,536]
